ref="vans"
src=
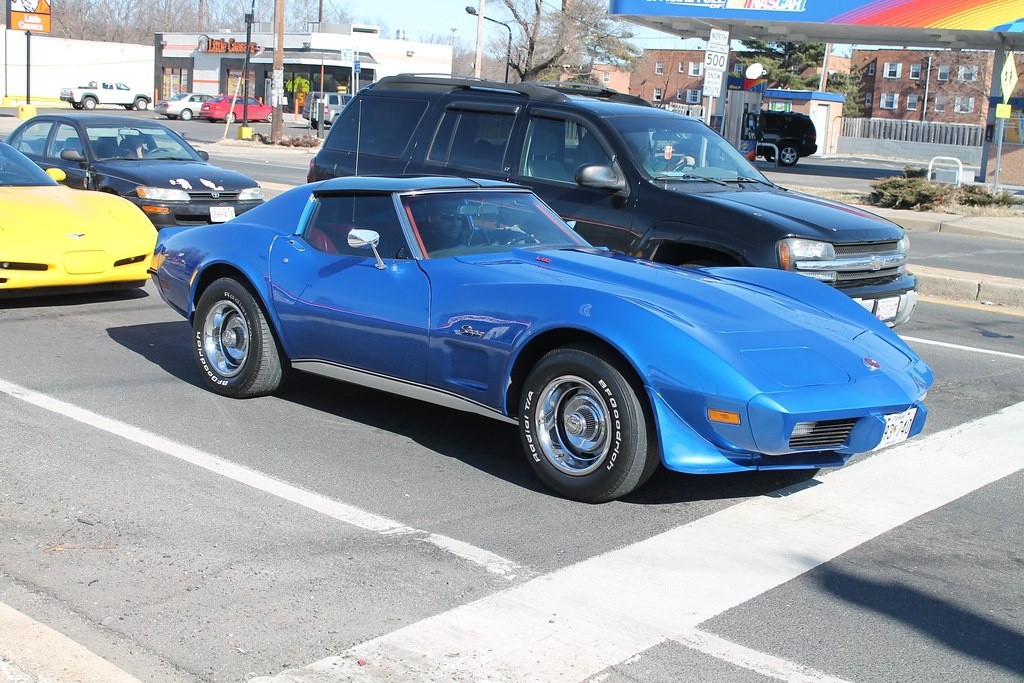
[303,92,356,130]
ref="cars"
[156,93,214,122]
[3,113,266,232]
[198,96,273,125]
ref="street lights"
[237,14,254,139]
[466,7,512,84]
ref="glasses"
[439,211,456,220]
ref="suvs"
[308,71,919,329]
[758,109,819,167]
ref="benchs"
[451,131,571,181]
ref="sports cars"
[0,142,161,298]
[146,178,932,504]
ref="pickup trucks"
[60,82,152,112]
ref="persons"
[117,130,158,159]
[218,92,224,103]
[402,194,540,260]
[606,124,695,190]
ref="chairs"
[19,137,118,158]
[310,221,406,259]
